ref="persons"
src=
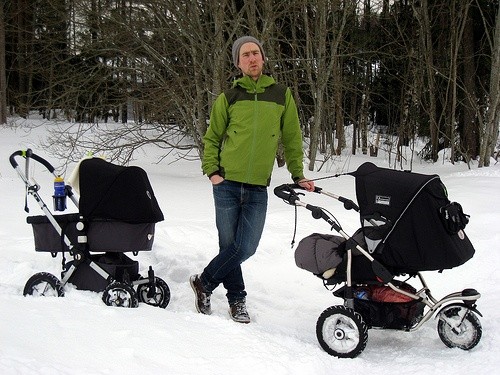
[189,36,315,324]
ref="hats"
[232,36,266,69]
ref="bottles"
[353,287,370,301]
[53,175,66,211]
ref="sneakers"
[189,273,212,315]
[227,297,251,323]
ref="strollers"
[8,152,171,308]
[274,162,485,358]
